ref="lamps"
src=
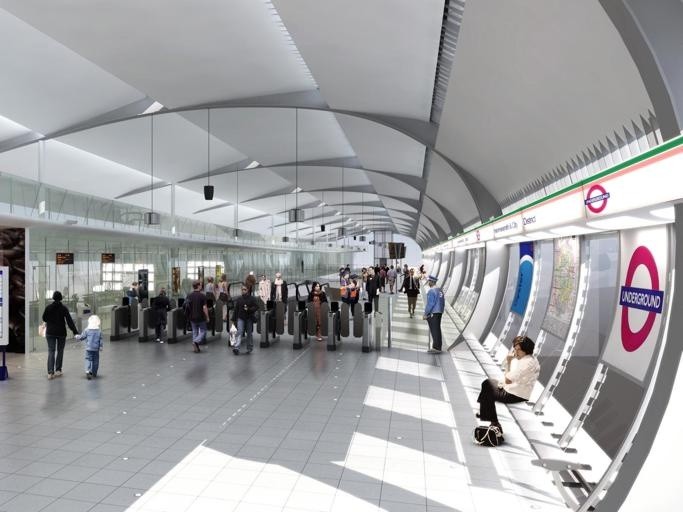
[337,166,348,239]
[233,172,242,238]
[359,191,366,242]
[204,109,214,199]
[288,108,305,222]
[283,194,289,242]
[145,116,160,225]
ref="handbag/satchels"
[471,425,504,448]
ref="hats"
[426,275,438,282]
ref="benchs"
[440,297,593,475]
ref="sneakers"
[191,341,200,353]
[233,349,240,355]
[47,370,63,380]
[426,348,442,354]
[86,371,97,380]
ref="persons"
[73,313,103,381]
[41,291,79,377]
[129,264,444,355]
[473,337,541,436]
